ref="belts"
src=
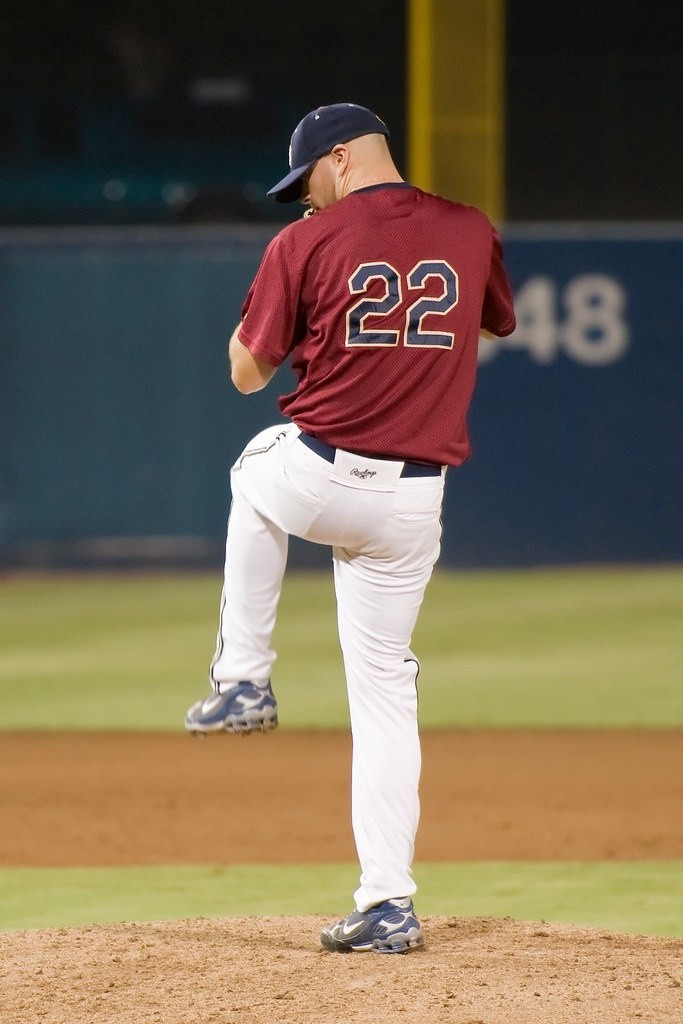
[298,432,441,478]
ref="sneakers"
[185,677,278,738]
[321,896,424,954]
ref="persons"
[182,102,517,953]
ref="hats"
[266,103,390,204]
